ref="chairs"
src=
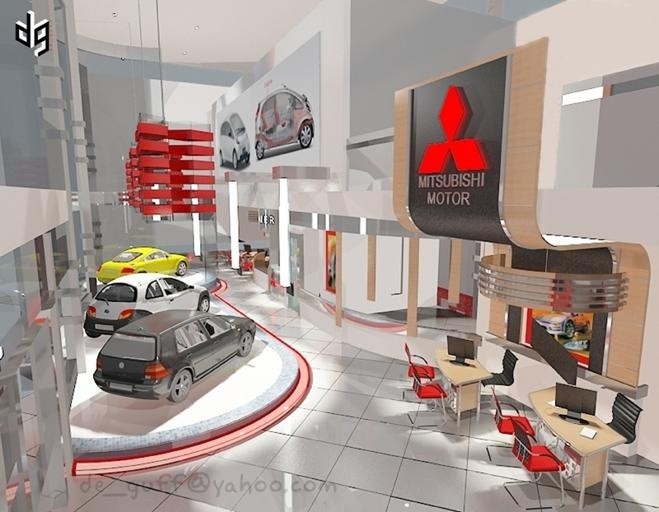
[402,342,644,511]
